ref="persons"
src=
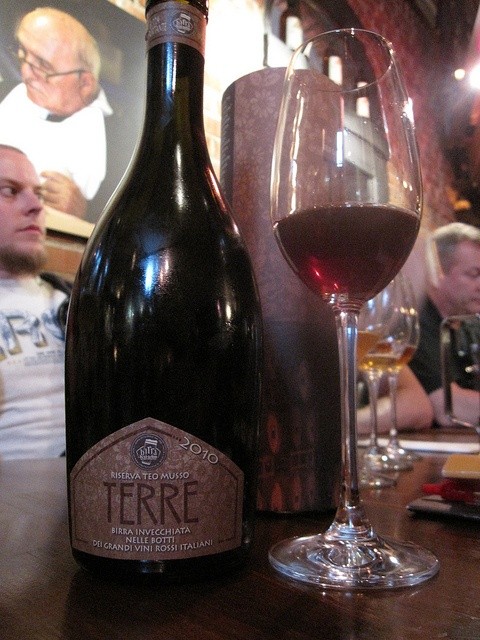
[355,354,432,442]
[1,143,68,462]
[417,223,480,428]
[0,5,138,225]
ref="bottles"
[66,0,261,598]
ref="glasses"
[5,45,87,82]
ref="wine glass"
[344,289,395,489]
[270,27,439,596]
[383,312,422,465]
[358,289,409,474]
